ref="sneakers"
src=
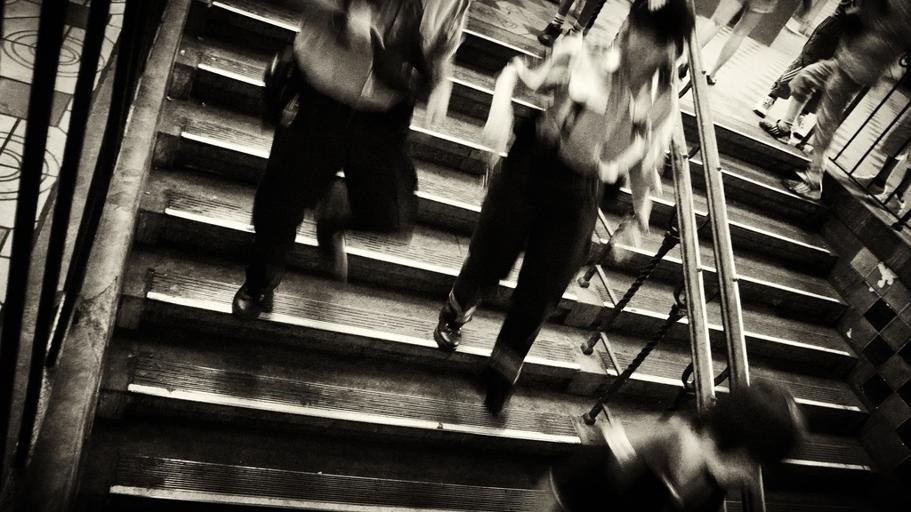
[794,114,809,138]
[760,120,794,144]
[851,171,886,194]
[874,189,907,217]
[784,172,824,200]
[753,94,774,117]
[537,18,563,47]
[563,28,581,38]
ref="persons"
[538,0,600,47]
[434,0,696,415]
[521,378,810,512]
[760,0,911,202]
[754,0,853,140]
[679,0,778,85]
[849,109,911,216]
[232,0,471,322]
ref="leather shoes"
[704,74,717,88]
[435,307,463,351]
[679,60,691,81]
[234,231,346,322]
[484,371,511,418]
